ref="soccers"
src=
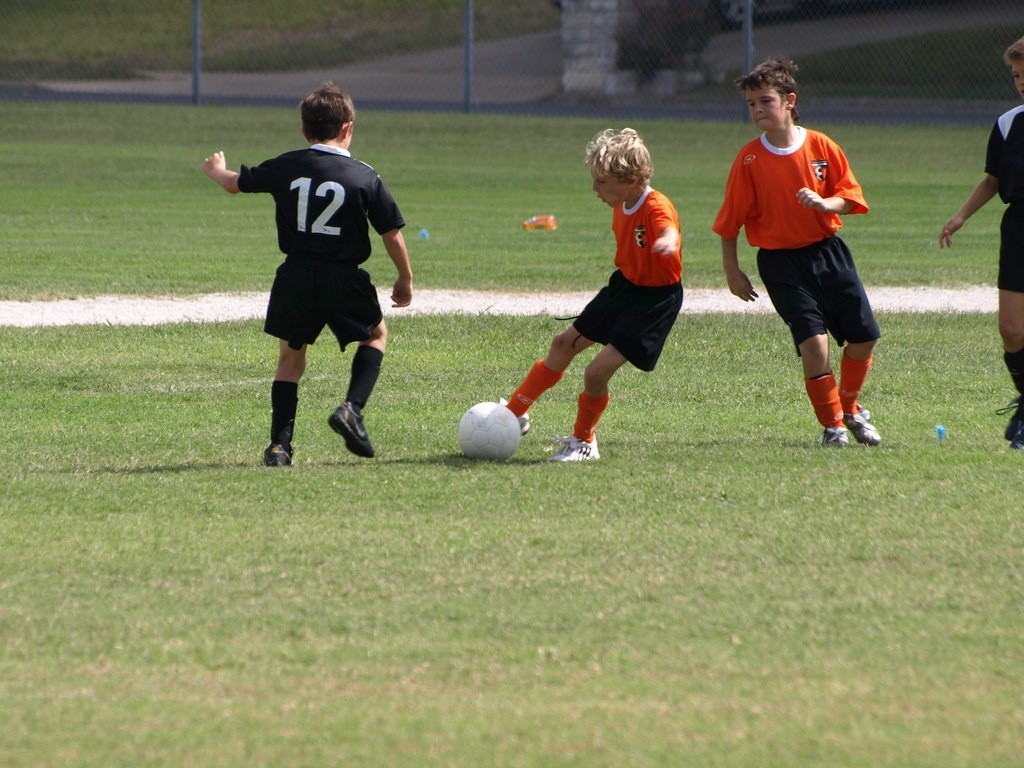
[458,401,521,462]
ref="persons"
[938,34,1024,448]
[712,57,881,446]
[201,79,413,466]
[498,127,683,462]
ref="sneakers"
[822,426,850,448]
[328,401,376,458]
[843,402,883,446]
[264,442,295,467]
[548,431,601,462]
[498,396,531,436]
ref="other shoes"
[996,395,1024,450]
